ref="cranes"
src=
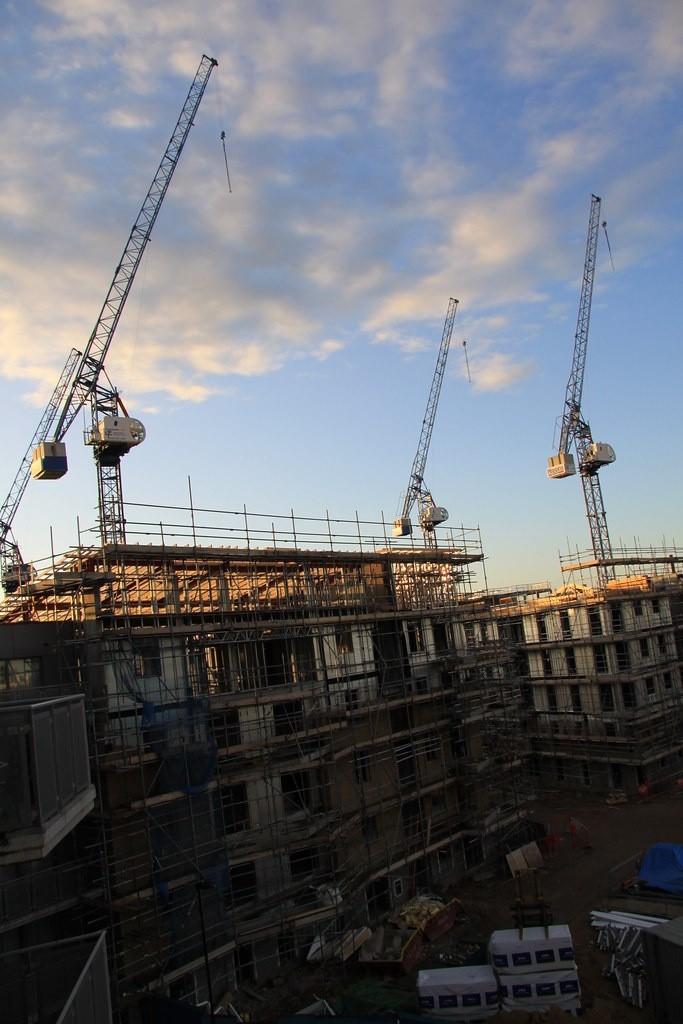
[1,53,237,566]
[548,192,625,588]
[392,295,472,547]
